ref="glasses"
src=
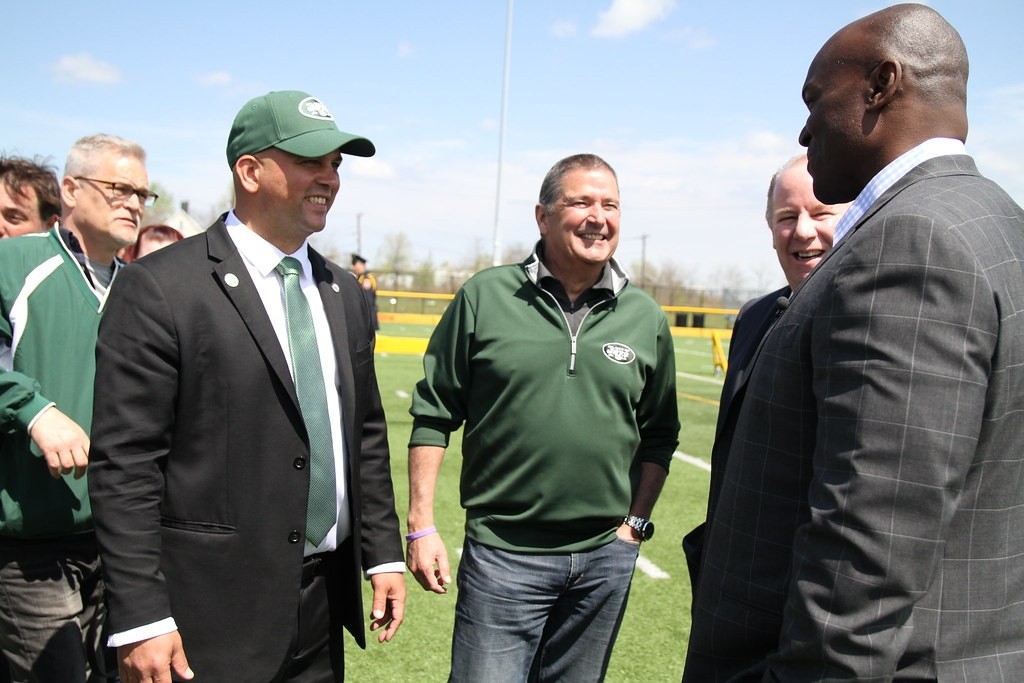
[75,176,158,208]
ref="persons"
[87,89,406,683]
[0,131,183,683]
[352,253,380,330]
[406,154,681,683]
[681,4,1024,683]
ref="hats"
[351,254,366,264]
[227,90,375,171]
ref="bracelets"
[405,527,437,541]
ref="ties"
[275,257,337,548]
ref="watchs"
[624,515,654,542]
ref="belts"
[301,536,353,590]
